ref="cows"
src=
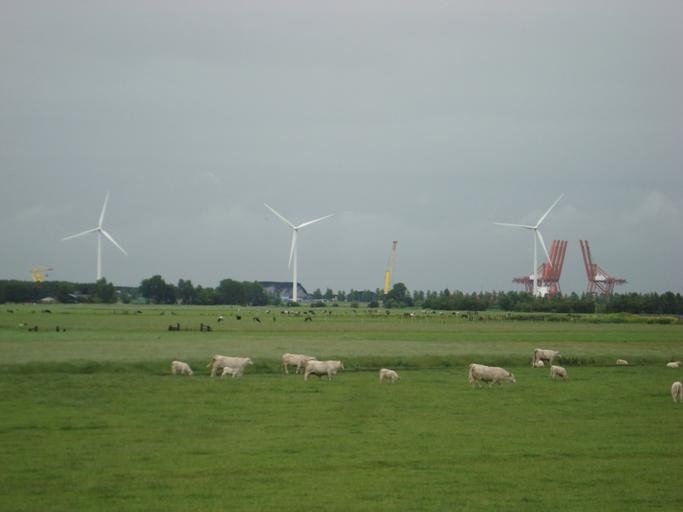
[171,360,193,376]
[206,354,253,377]
[468,363,516,389]
[531,348,562,367]
[671,381,683,403]
[550,365,568,382]
[666,361,682,368]
[280,352,345,382]
[616,359,628,366]
[379,368,400,385]
